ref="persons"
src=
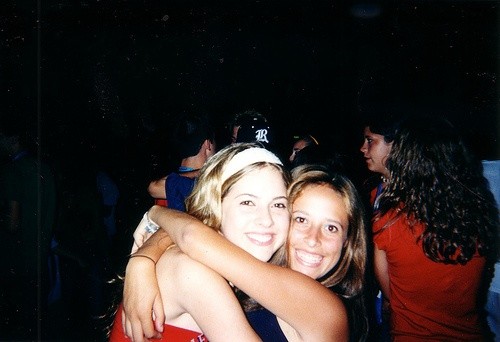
[360,110,428,342]
[122,165,366,342]
[148,110,344,215]
[372,113,500,342]
[109,142,292,342]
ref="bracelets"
[127,253,156,265]
[142,212,160,235]
